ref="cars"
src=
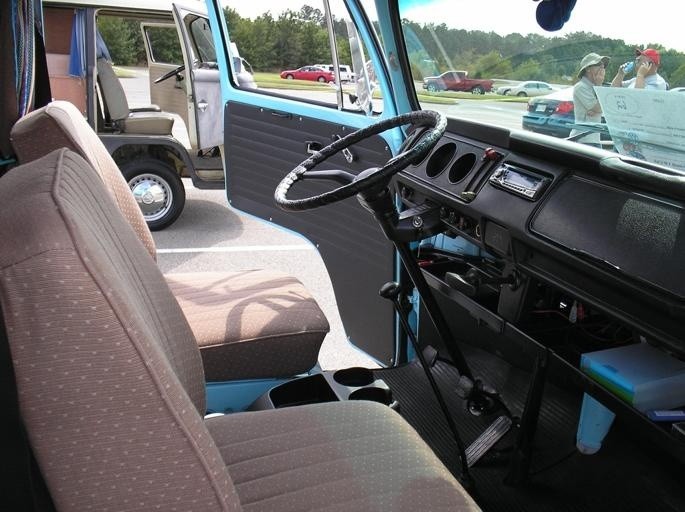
[282,66,334,85]
[497,80,615,141]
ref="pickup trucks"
[423,70,495,95]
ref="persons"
[569,52,611,148]
[611,49,666,90]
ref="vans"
[314,64,352,82]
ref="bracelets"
[637,74,645,78]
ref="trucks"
[39,0,264,228]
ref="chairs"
[97,58,174,134]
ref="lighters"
[646,409,685,421]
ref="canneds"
[622,62,635,74]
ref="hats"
[578,53,611,79]
[636,48,660,66]
